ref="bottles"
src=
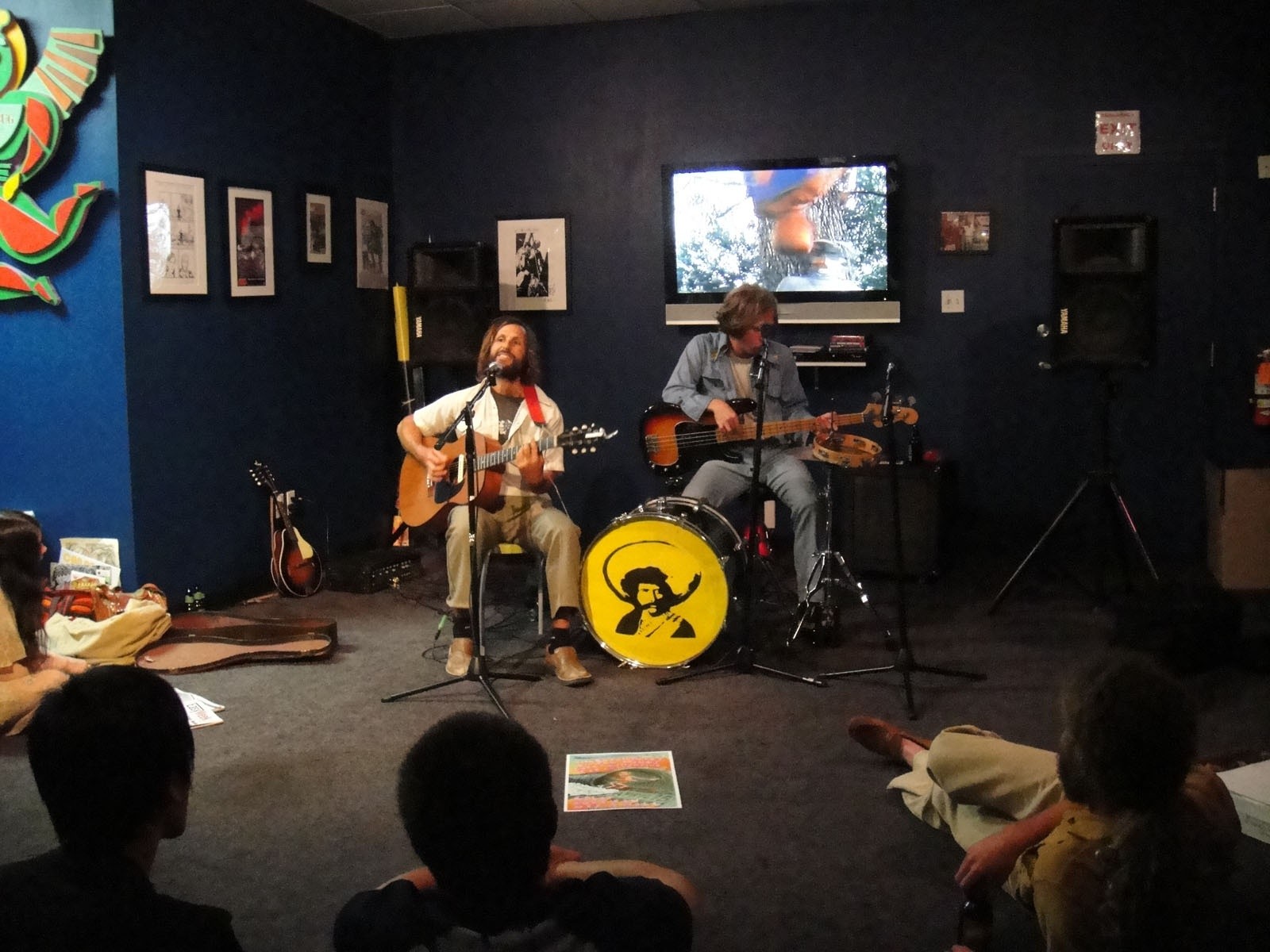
[958,885,999,952]
[1254,349,1270,426]
[907,425,922,462]
[184,585,206,613]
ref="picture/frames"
[227,186,275,297]
[306,193,332,263]
[144,168,208,295]
[355,197,389,290]
[492,212,575,317]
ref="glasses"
[752,319,783,339]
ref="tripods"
[655,343,826,685]
[816,379,990,721]
[383,377,540,721]
[985,379,1168,612]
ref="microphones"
[485,362,503,379]
[884,356,899,375]
[760,324,778,354]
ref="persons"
[661,284,839,604]
[396,315,595,687]
[0,508,173,737]
[332,712,702,952]
[0,666,245,952]
[847,660,1242,952]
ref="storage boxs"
[1201,463,1270,587]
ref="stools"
[479,543,544,656]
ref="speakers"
[836,458,955,583]
[1051,215,1152,369]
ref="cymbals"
[578,497,751,669]
[812,431,884,469]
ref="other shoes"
[847,717,933,763]
[798,601,816,629]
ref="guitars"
[249,460,323,599]
[397,423,621,527]
[637,397,918,476]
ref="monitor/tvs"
[660,155,901,302]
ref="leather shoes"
[546,643,592,685]
[446,636,477,676]
